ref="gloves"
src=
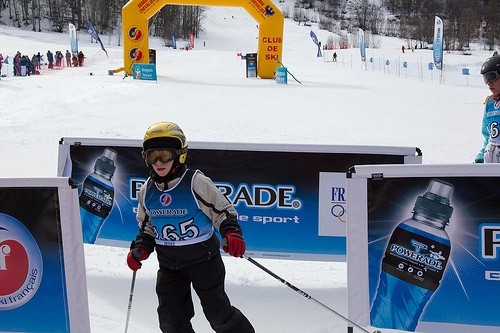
[223,230,245,257]
[127,247,149,271]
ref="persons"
[472,55,500,163]
[127,122,255,333]
[0,50,84,76]
[402,46,404,53]
[333,52,337,62]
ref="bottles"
[370,179,454,332]
[79,149,117,244]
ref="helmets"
[481,55,500,75]
[143,122,186,154]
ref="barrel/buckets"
[276,67,287,84]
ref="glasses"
[148,148,182,164]
[484,70,500,85]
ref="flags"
[359,28,364,57]
[310,31,318,46]
[190,31,194,48]
[433,16,443,69]
[69,23,77,53]
[172,31,176,49]
